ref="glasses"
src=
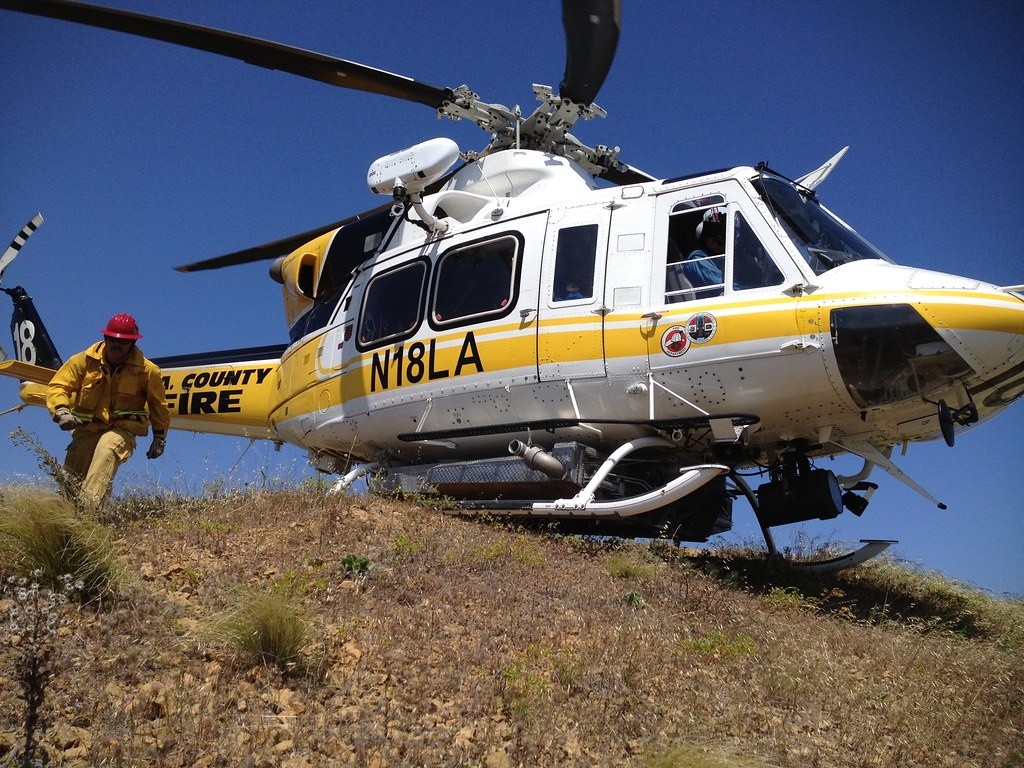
[107,336,132,345]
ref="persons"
[45,314,170,522]
[553,269,583,302]
[682,221,739,299]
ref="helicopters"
[1,0,1024,561]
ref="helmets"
[101,312,143,340]
[694,206,741,247]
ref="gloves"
[146,437,167,459]
[52,409,83,432]
